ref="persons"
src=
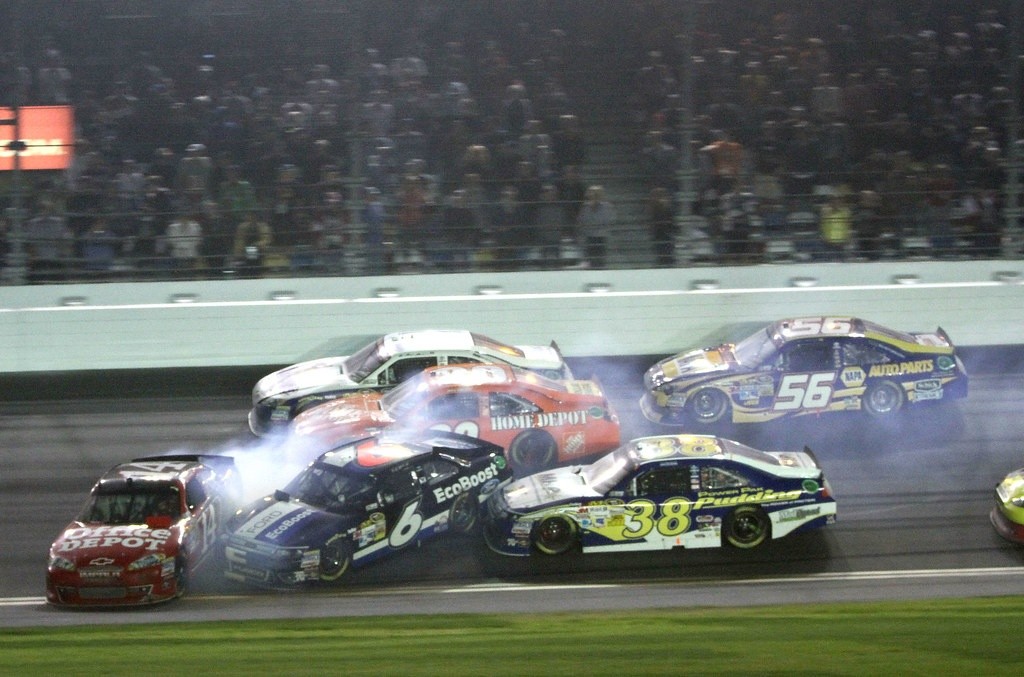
[0,0,1024,288]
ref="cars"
[214,427,517,594]
[247,330,577,440]
[481,435,839,559]
[45,453,240,609]
[639,314,970,427]
[989,465,1024,546]
[287,362,621,478]
